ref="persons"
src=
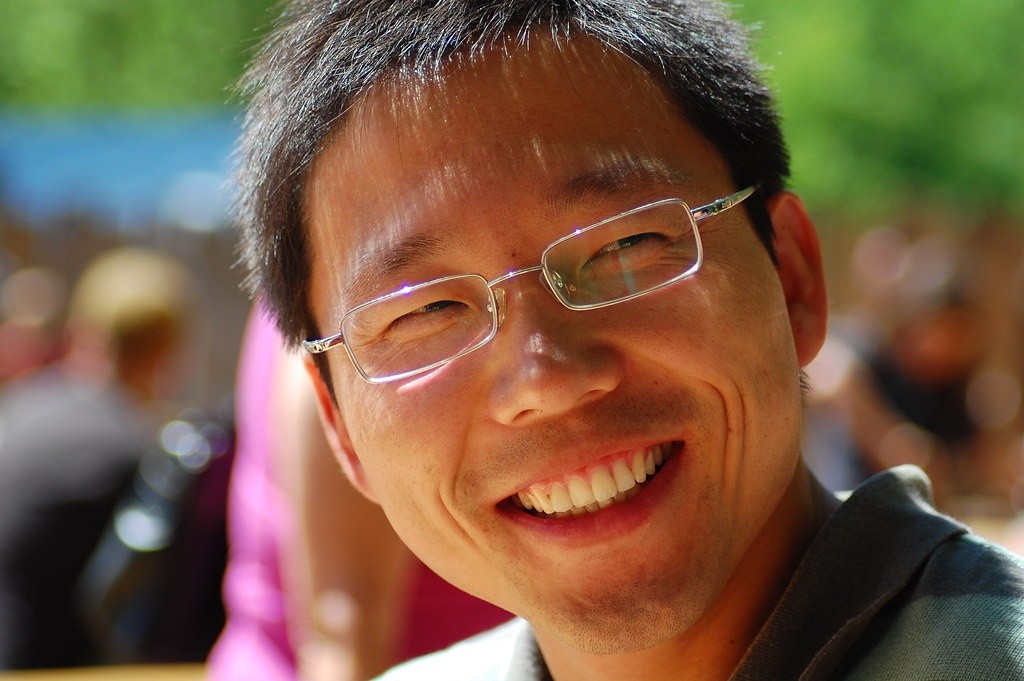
[0,246,231,671]
[232,1,1024,681]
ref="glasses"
[302,185,758,383]
